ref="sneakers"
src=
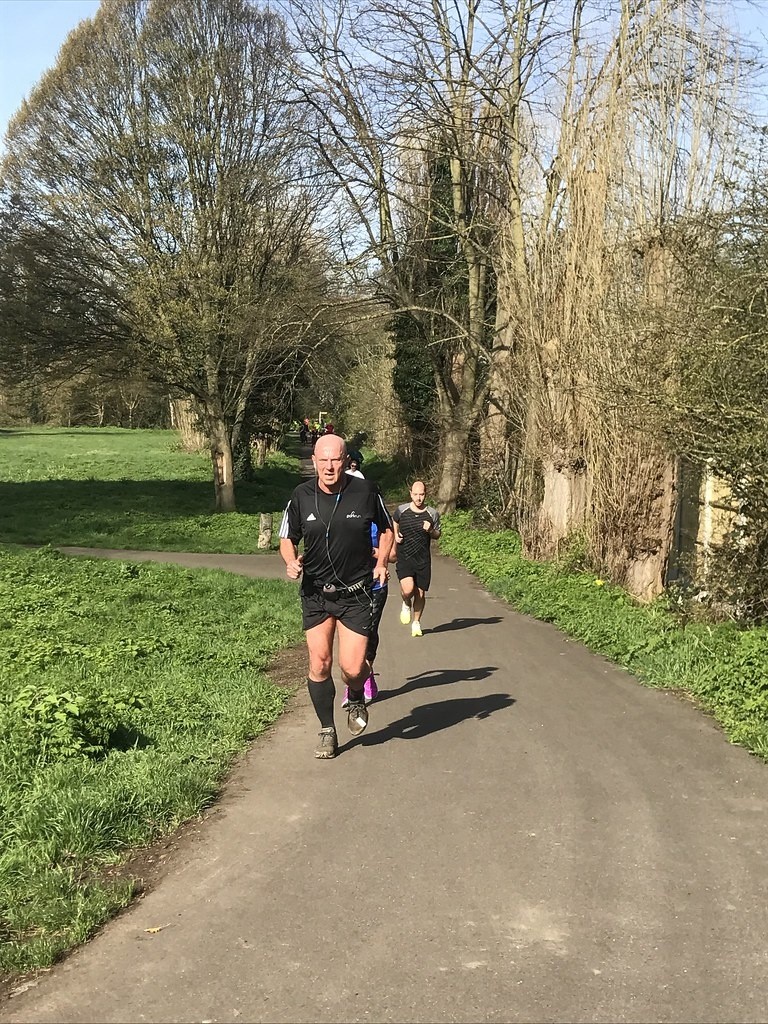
[314,727,338,759]
[341,684,349,708]
[412,621,422,637]
[364,668,380,699]
[400,600,412,624]
[347,695,368,735]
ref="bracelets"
[429,529,434,534]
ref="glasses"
[351,465,356,467]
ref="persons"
[392,481,441,635]
[295,414,397,708]
[278,434,395,759]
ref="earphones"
[314,461,317,468]
